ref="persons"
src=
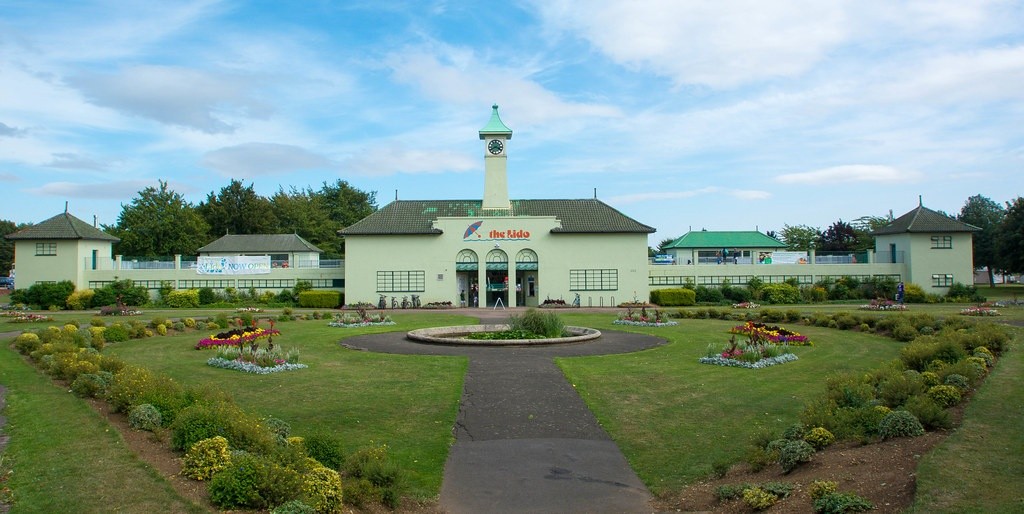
[850,255,857,263]
[896,281,904,305]
[757,251,773,265]
[733,247,739,265]
[715,248,728,266]
[460,289,466,307]
[672,260,676,265]
[469,284,479,308]
[795,257,808,266]
[272,258,290,269]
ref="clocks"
[488,139,503,155]
